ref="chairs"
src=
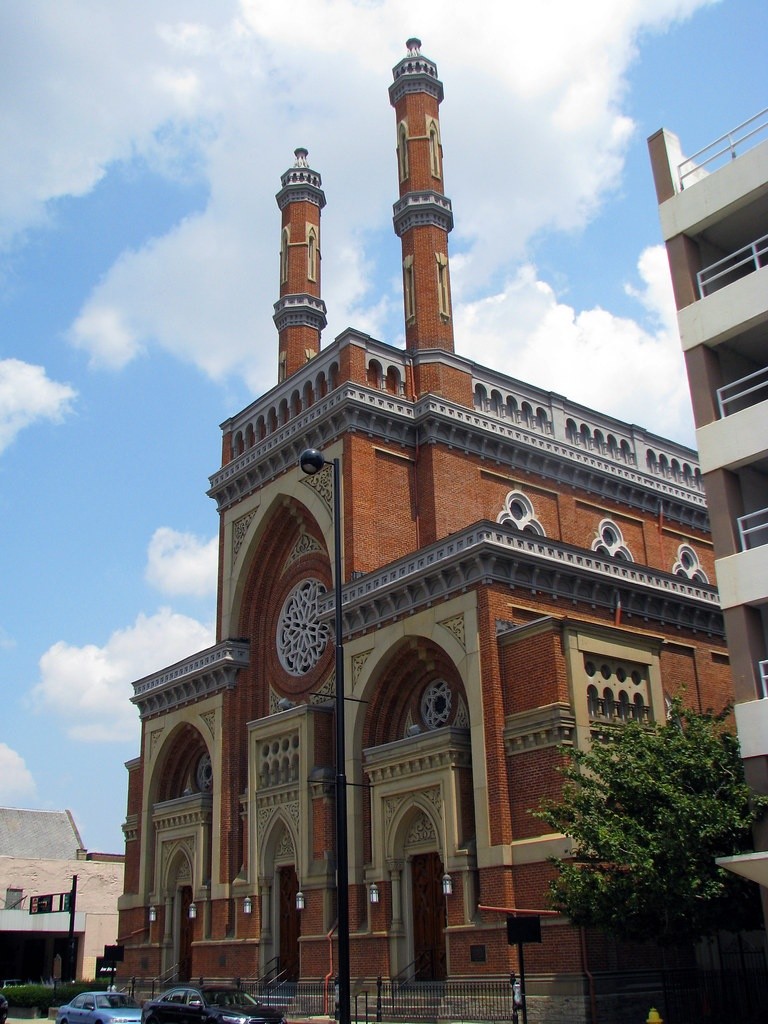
[109,998,115,1008]
[191,995,202,1001]
[172,995,182,1003]
[216,992,241,1005]
[83,1002,94,1009]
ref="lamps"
[241,896,252,914]
[188,902,196,919]
[442,871,453,895]
[184,789,192,797]
[148,904,157,922]
[279,698,292,711]
[295,890,305,910]
[369,881,380,904]
[408,724,424,736]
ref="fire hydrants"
[647,1008,663,1024]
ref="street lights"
[298,445,356,1024]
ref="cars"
[140,984,284,1024]
[2,979,26,989]
[53,991,143,1024]
